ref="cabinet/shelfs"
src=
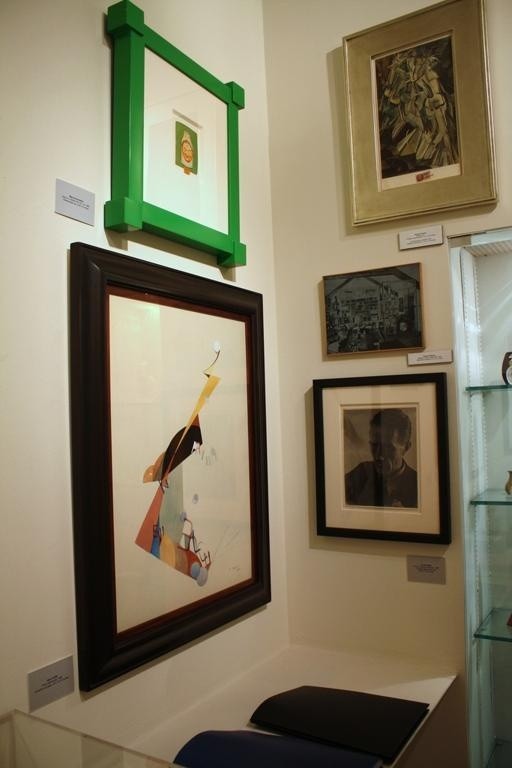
[447,225,512,768]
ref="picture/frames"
[340,0,497,230]
[321,260,424,358]
[104,0,246,267]
[312,371,451,544]
[66,240,272,693]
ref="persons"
[345,410,417,510]
